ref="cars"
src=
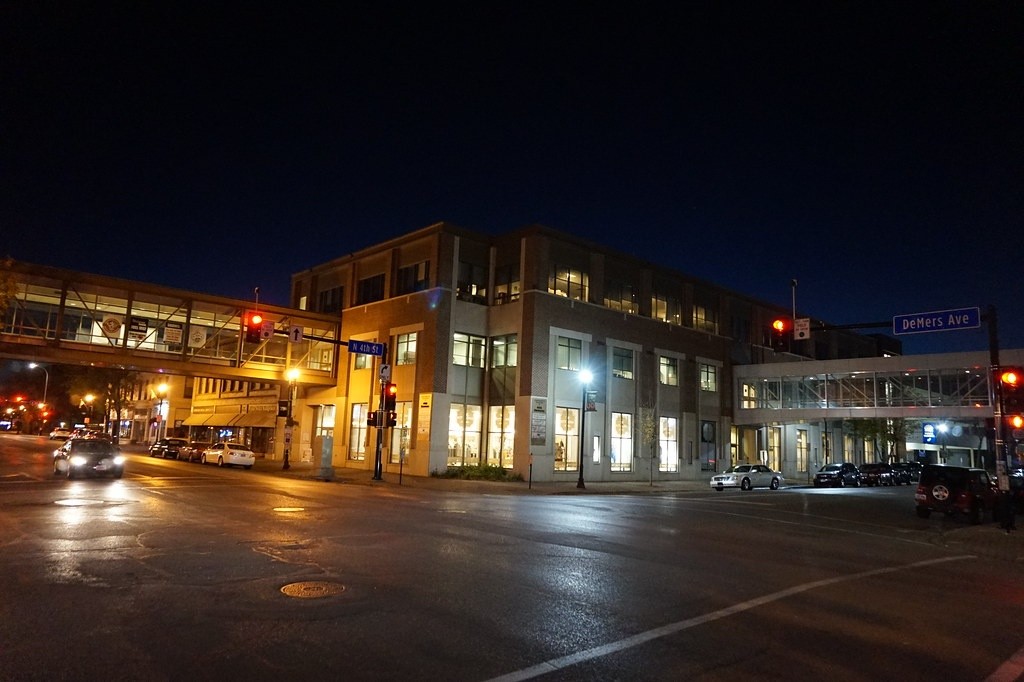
[201,442,256,470]
[709,464,784,491]
[148,438,189,458]
[52,440,118,480]
[812,461,863,488]
[857,461,922,487]
[176,443,211,462]
[49,427,113,445]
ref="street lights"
[28,363,49,405]
[576,368,594,488]
[791,277,798,320]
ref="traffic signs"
[378,364,391,378]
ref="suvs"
[914,463,1001,525]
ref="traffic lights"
[1008,415,1024,430]
[380,384,396,411]
[1001,371,1020,386]
[771,318,793,338]
[367,411,377,426]
[386,412,397,429]
[246,311,263,344]
